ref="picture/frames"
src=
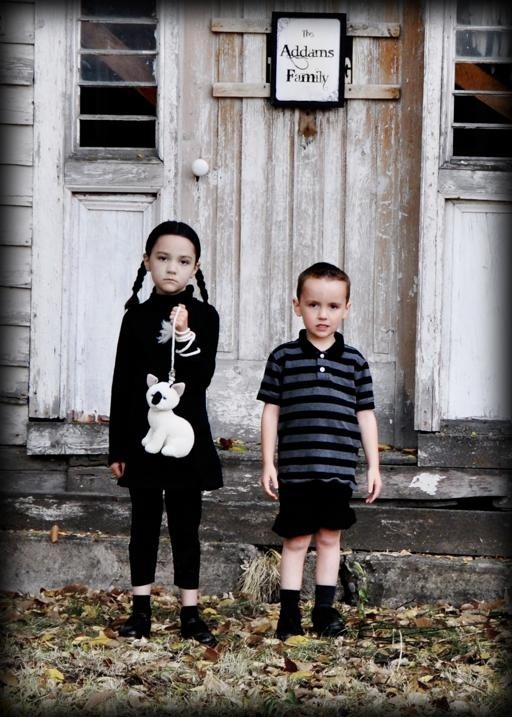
[269,11,345,110]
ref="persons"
[106,218,222,650]
[255,262,385,638]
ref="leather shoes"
[182,618,216,647]
[312,606,345,635]
[276,616,303,641]
[118,617,151,638]
